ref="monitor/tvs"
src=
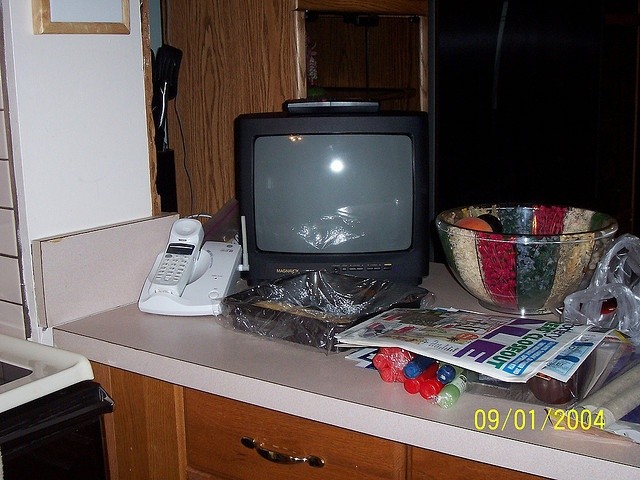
[234,111,429,295]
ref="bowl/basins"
[434,203,620,317]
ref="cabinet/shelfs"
[184,361,406,480]
[161,2,429,221]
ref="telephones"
[137,218,250,317]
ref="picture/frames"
[34,0,131,33]
